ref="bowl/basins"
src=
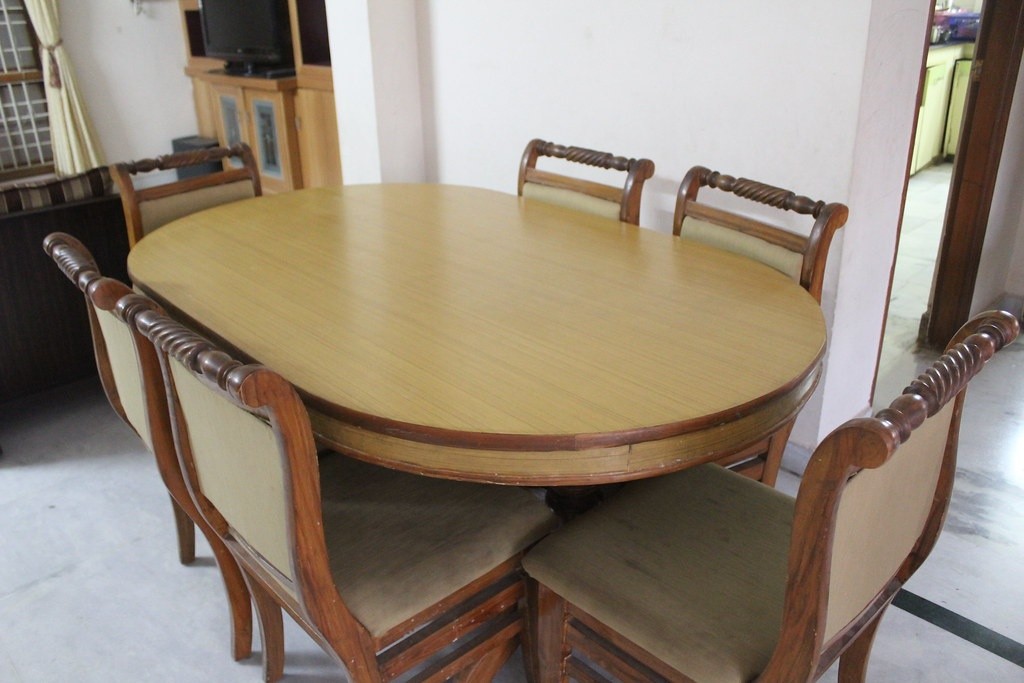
[930,26,941,44]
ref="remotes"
[266,68,296,79]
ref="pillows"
[0,165,114,215]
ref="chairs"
[115,291,546,683]
[515,139,658,225]
[40,235,204,561]
[520,311,1024,682]
[110,143,265,249]
[668,167,849,489]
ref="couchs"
[0,194,129,404]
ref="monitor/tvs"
[199,0,294,76]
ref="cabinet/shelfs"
[201,79,303,194]
[911,47,975,177]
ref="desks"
[132,181,826,504]
